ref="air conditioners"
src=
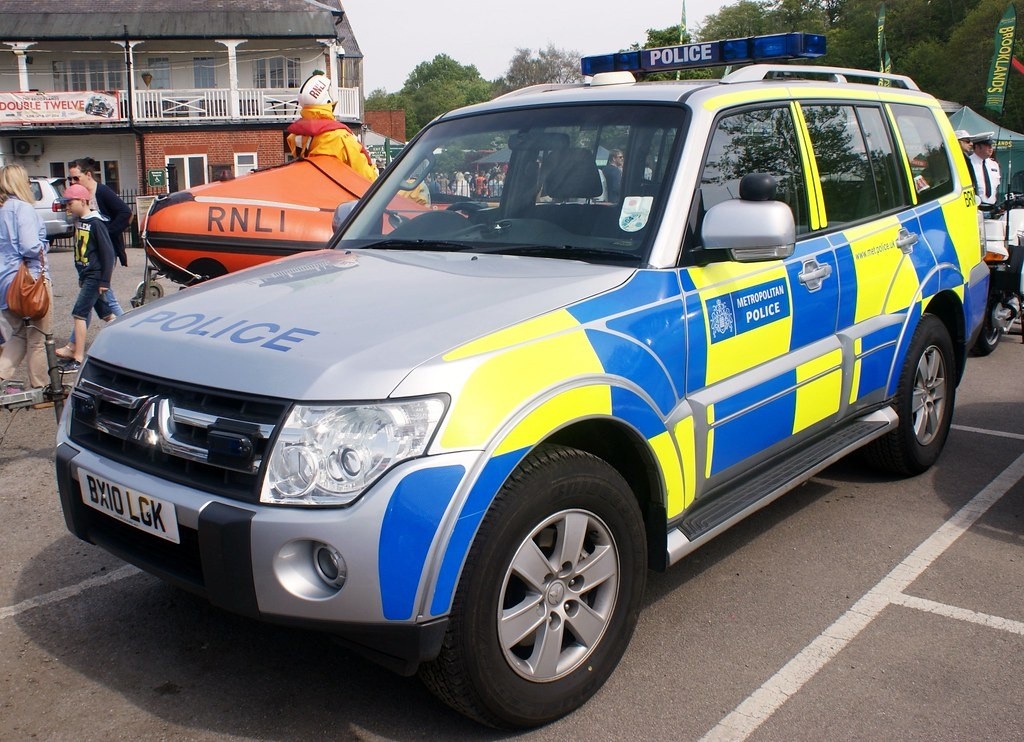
[13,137,44,157]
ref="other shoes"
[2,385,29,394]
[58,358,81,373]
[54,344,75,358]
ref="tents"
[949,106,1024,203]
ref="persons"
[970,132,1002,206]
[55,157,134,358]
[954,130,979,195]
[603,148,653,203]
[55,184,118,373]
[0,164,66,408]
[424,167,505,198]
[288,75,427,204]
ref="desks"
[266,100,298,121]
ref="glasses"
[961,139,972,143]
[66,175,81,181]
[28,178,32,185]
[616,155,624,159]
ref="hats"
[954,130,976,140]
[973,131,995,143]
[56,184,90,201]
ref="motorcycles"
[970,170,1024,357]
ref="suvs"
[52,27,990,732]
[27,176,76,244]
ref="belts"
[977,205,994,211]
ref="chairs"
[519,146,620,236]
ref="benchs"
[199,99,259,121]
[120,100,189,123]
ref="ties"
[982,158,992,198]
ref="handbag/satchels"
[5,249,49,321]
